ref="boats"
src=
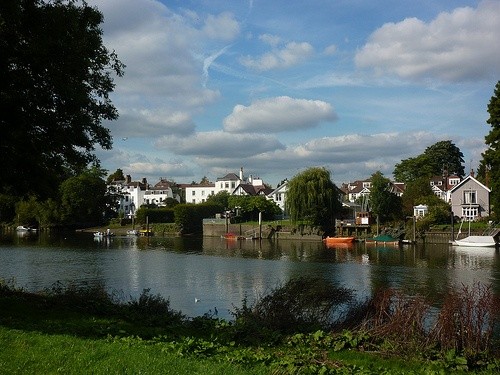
[14,226,40,231]
[366,234,400,244]
[449,235,496,247]
[321,235,354,242]
[94,231,114,236]
[127,226,153,237]
[219,232,244,240]
